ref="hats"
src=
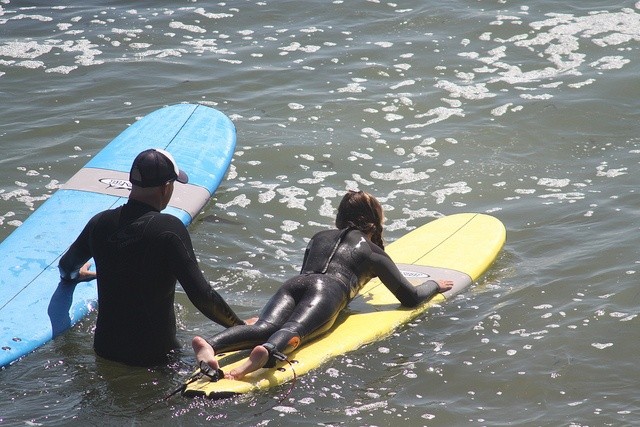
[129,149,188,188]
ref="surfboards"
[181,212,507,399]
[0,102,236,373]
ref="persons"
[57,149,259,372]
[192,190,455,379]
